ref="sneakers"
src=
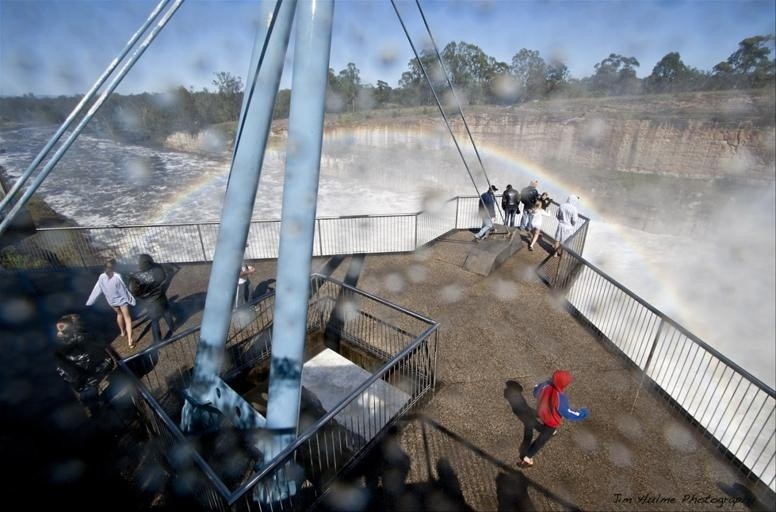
[473,233,571,262]
[519,456,538,468]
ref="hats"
[488,184,498,191]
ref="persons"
[474,179,579,258]
[234,244,261,312]
[85,258,136,349]
[44,310,149,480]
[522,370,589,467]
[128,253,175,345]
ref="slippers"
[117,330,139,350]
